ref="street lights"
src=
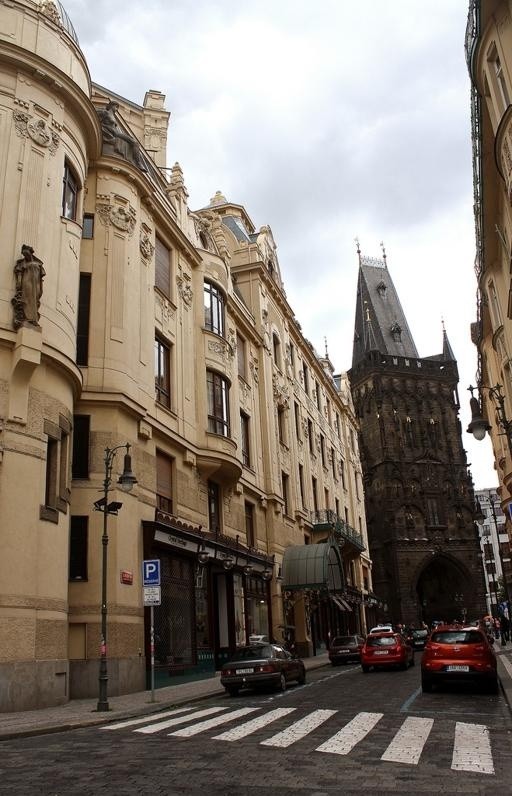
[463,383,512,459]
[475,523,499,606]
[474,490,510,622]
[94,441,138,711]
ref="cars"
[248,635,268,645]
[360,632,414,672]
[419,624,498,695]
[219,643,306,697]
[370,626,399,635]
[329,634,364,665]
[407,628,429,650]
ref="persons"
[282,629,296,656]
[14,243,47,328]
[395,615,511,642]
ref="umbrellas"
[278,625,296,629]
